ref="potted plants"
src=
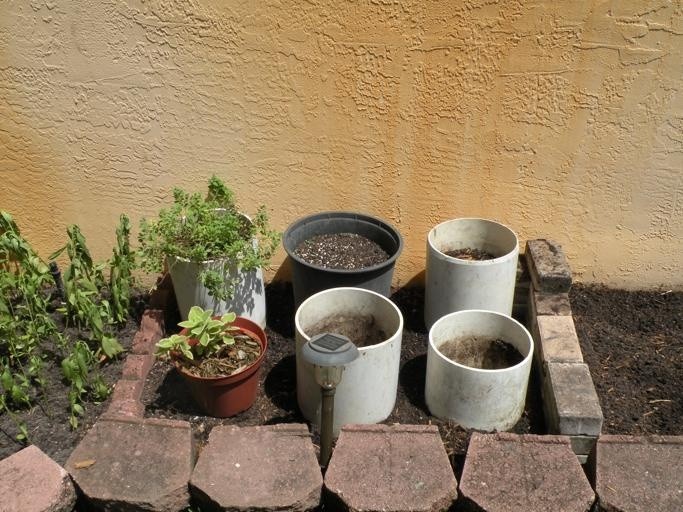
[132,173,284,418]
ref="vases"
[423,217,520,332]
[294,286,403,437]
[285,213,404,306]
[425,308,537,435]
